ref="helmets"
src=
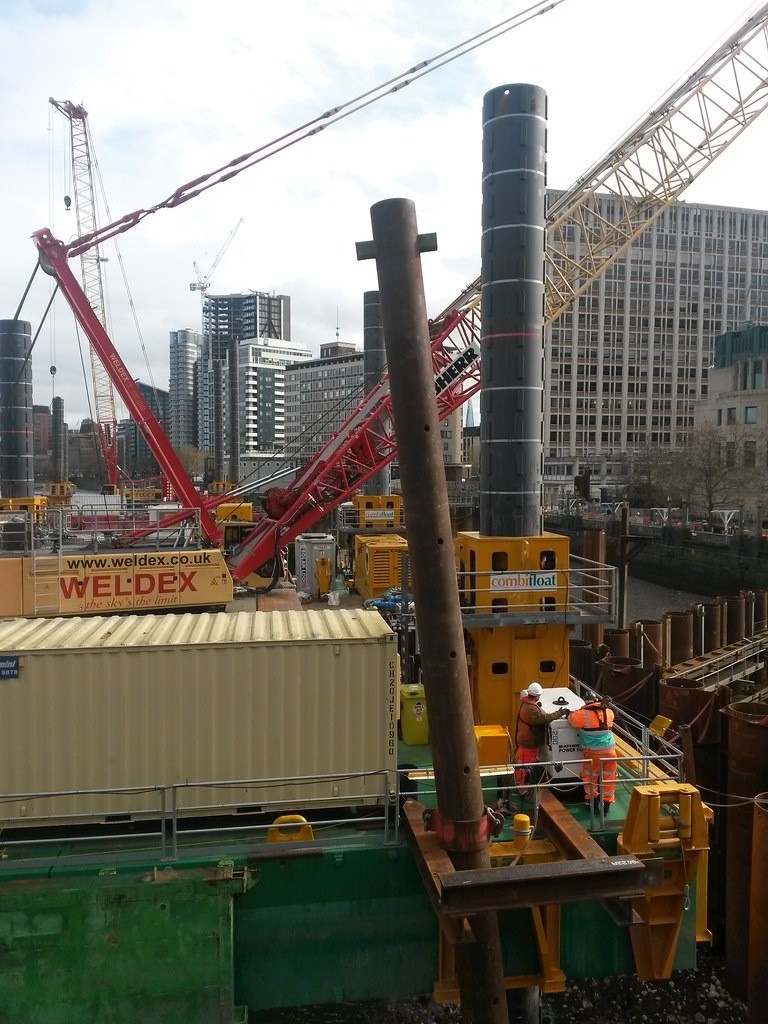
[527,682,542,696]
[585,692,596,700]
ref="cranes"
[210,2,768,605]
[45,92,122,497]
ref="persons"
[567,692,616,818]
[516,683,571,804]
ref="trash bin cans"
[703,526,712,532]
[400,683,430,745]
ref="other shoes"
[585,799,599,813]
[604,802,610,816]
